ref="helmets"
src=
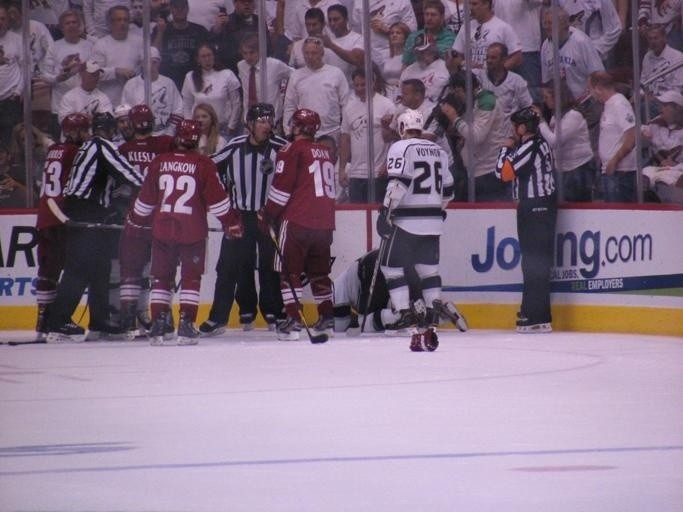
[246,103,275,121]
[286,109,319,135]
[397,113,423,138]
[176,119,201,146]
[62,105,153,137]
[510,109,539,124]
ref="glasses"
[304,38,319,45]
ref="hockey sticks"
[137,279,184,329]
[266,221,328,343]
[360,237,385,338]
[45,198,223,232]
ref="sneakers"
[200,313,334,331]
[516,311,550,326]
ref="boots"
[385,299,457,330]
[36,302,200,336]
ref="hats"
[140,46,161,62]
[413,33,435,51]
[655,90,683,107]
[78,59,103,73]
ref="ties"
[249,67,257,109]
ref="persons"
[1,1,682,353]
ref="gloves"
[124,210,147,242]
[377,207,394,239]
[223,211,244,239]
[257,205,279,236]
[410,328,438,351]
